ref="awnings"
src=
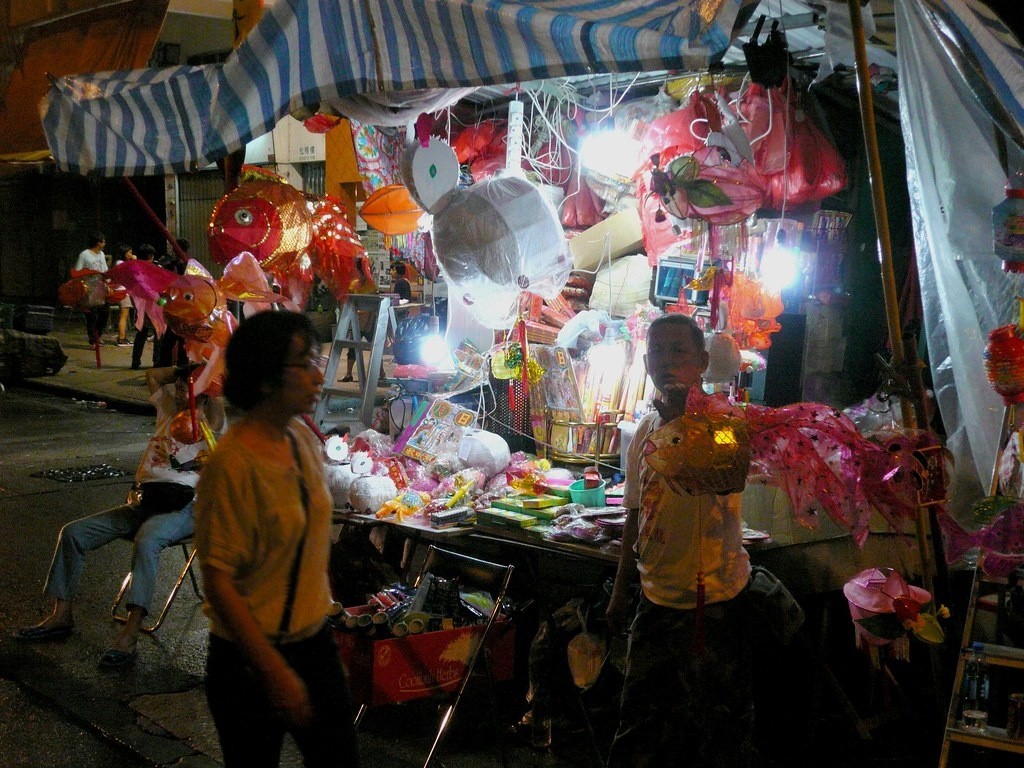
[1,0,176,165]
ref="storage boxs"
[335,594,517,707]
[744,473,932,586]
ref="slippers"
[22,623,73,637]
[99,648,139,668]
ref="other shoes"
[118,339,134,346]
[336,374,353,383]
[90,343,96,349]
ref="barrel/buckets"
[568,478,606,507]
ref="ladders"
[938,404,1024,768]
[313,294,419,429]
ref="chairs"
[112,532,201,631]
[350,543,518,768]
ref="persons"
[111,238,189,369]
[606,314,753,768]
[384,262,411,363]
[337,254,387,382]
[195,310,363,768]
[75,229,111,350]
[12,362,228,670]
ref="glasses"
[285,355,328,370]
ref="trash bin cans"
[0,304,55,335]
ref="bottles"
[961,642,991,732]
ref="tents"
[37,0,917,440]
